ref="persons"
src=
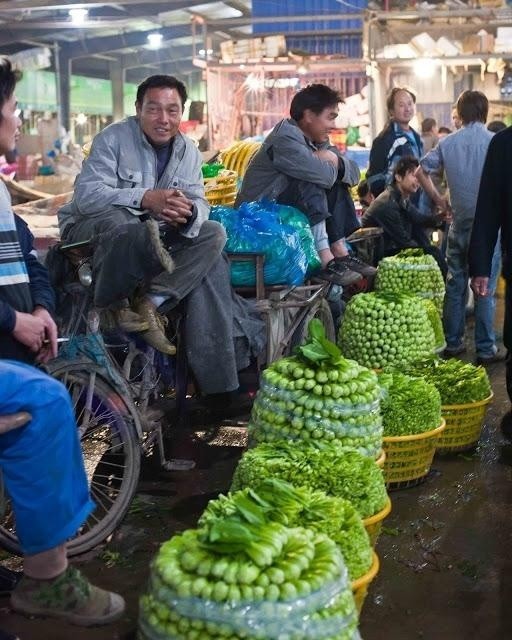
[361,156,450,285]
[417,102,509,260]
[233,83,380,288]
[56,74,229,356]
[462,123,511,443]
[412,89,508,367]
[355,179,376,215]
[1,57,127,628]
[365,86,433,234]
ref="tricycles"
[0,252,337,558]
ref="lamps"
[64,8,89,29]
[147,30,166,52]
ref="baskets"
[374,451,386,468]
[438,389,495,456]
[201,417,446,624]
[383,417,446,482]
[362,497,392,547]
[351,551,380,616]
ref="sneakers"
[339,256,377,276]
[477,347,509,367]
[131,298,177,356]
[316,257,363,287]
[97,296,150,335]
[8,564,126,629]
[439,348,468,362]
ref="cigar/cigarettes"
[43,337,73,345]
